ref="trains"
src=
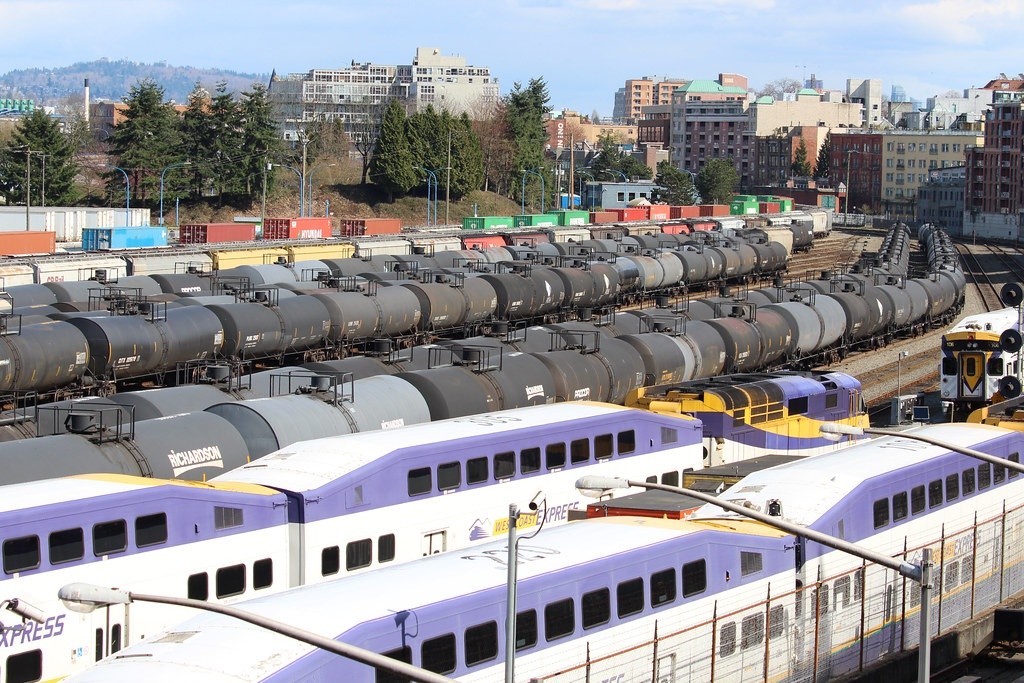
[0,373,871,682]
[46,396,1023,683]
[940,307,1024,416]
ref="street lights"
[606,169,627,208]
[160,162,192,227]
[600,170,616,183]
[578,171,594,212]
[412,167,437,226]
[427,167,453,227]
[520,169,544,214]
[575,475,934,683]
[818,424,1024,473]
[309,163,336,217]
[273,164,303,217]
[568,166,591,209]
[58,582,458,683]
[97,164,129,227]
[522,167,545,215]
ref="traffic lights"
[1001,282,1023,307]
[999,329,1023,353]
[1000,375,1022,399]
